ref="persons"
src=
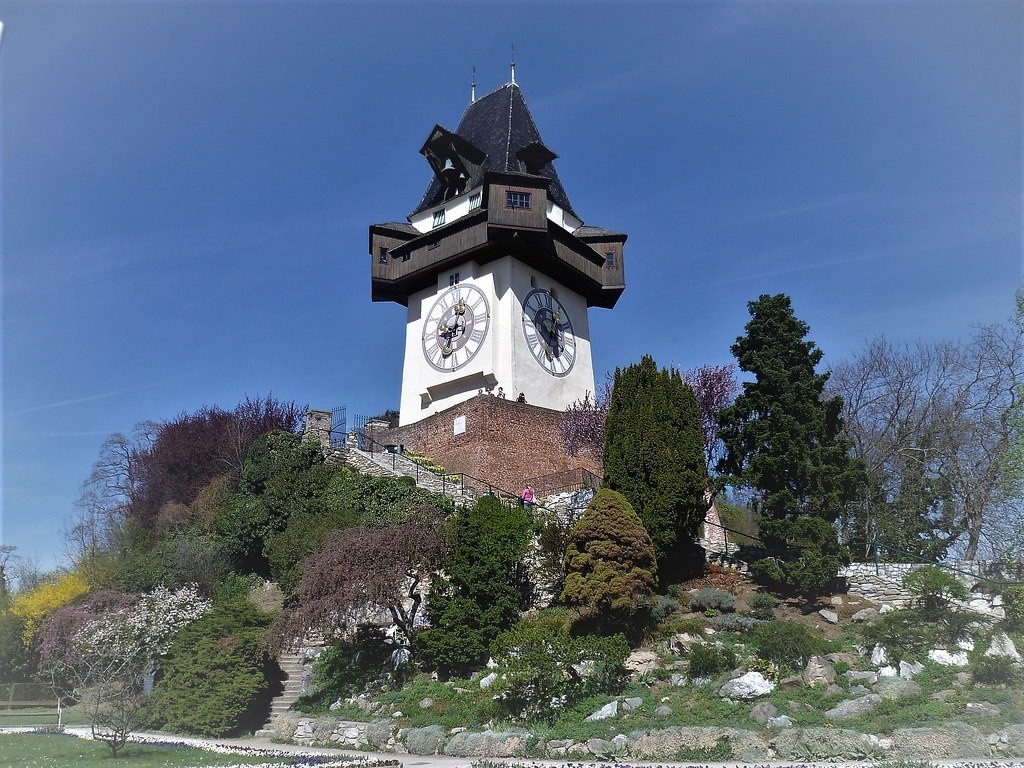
[487,387,494,397]
[517,392,527,403]
[497,387,506,399]
[478,389,483,395]
[521,483,536,508]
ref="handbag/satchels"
[532,490,536,504]
